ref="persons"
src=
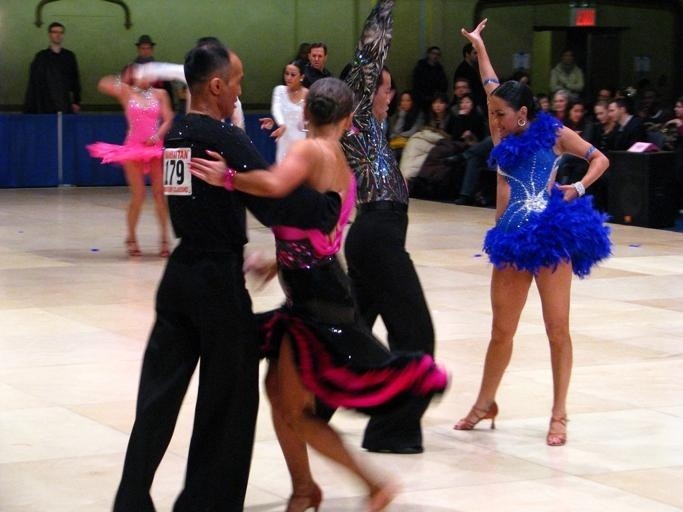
[409,94,481,197]
[270,60,309,178]
[134,37,243,130]
[426,91,458,140]
[454,18,611,445]
[563,102,594,145]
[594,99,620,152]
[548,51,585,100]
[188,79,399,512]
[451,79,483,116]
[383,76,397,137]
[411,46,448,114]
[122,35,175,116]
[24,23,82,114]
[639,91,662,123]
[86,58,175,259]
[259,42,334,130]
[454,43,486,111]
[389,89,427,158]
[536,93,548,113]
[552,90,571,121]
[608,100,649,150]
[314,0,435,454]
[439,120,494,204]
[663,97,683,137]
[296,43,313,63]
[113,38,347,512]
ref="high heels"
[161,241,169,257]
[453,400,498,431]
[286,483,322,512]
[547,413,570,446]
[124,239,142,257]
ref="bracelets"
[223,170,236,190]
[574,182,585,196]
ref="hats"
[134,34,155,46]
